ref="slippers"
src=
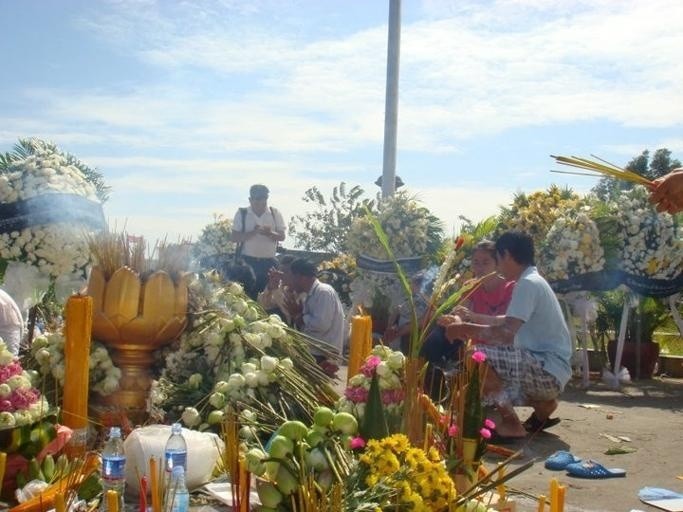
[523,412,560,432]
[544,449,582,471]
[565,460,627,479]
[484,427,517,445]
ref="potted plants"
[597,294,672,379]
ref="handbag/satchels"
[228,258,256,286]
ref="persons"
[379,270,434,355]
[443,231,574,445]
[278,257,345,366]
[433,239,519,348]
[254,255,307,332]
[229,183,286,301]
[644,167,682,215]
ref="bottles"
[100,426,125,512]
[164,423,187,487]
[166,465,189,512]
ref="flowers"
[498,182,683,289]
